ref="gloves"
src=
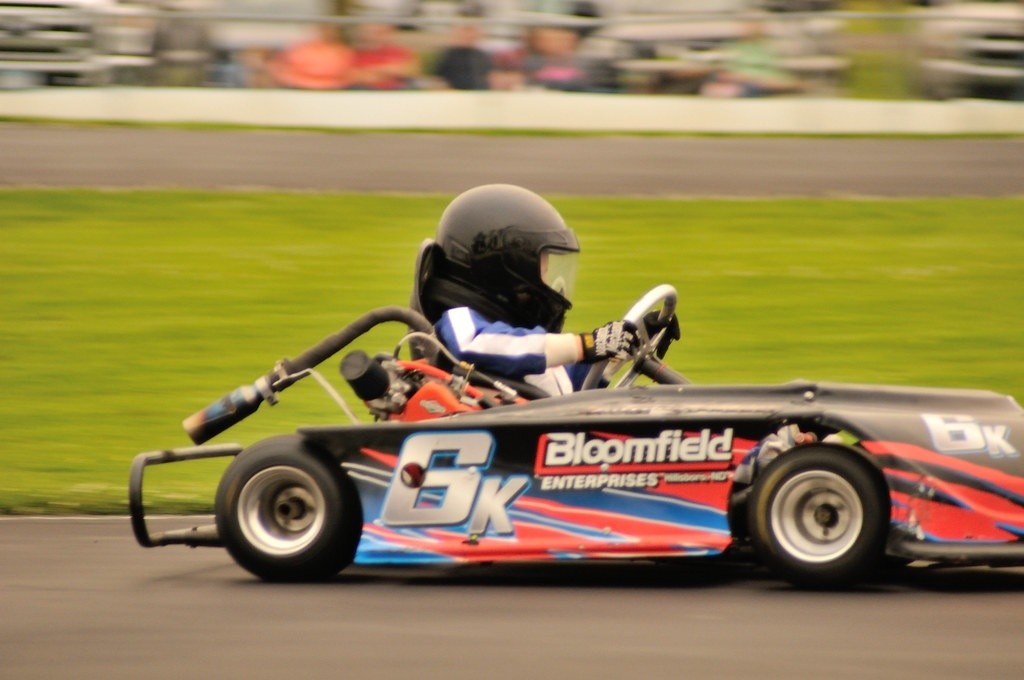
[642,310,680,360]
[577,319,639,364]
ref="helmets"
[432,183,580,334]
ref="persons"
[408,183,804,484]
[270,23,615,93]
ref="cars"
[908,0,1024,103]
